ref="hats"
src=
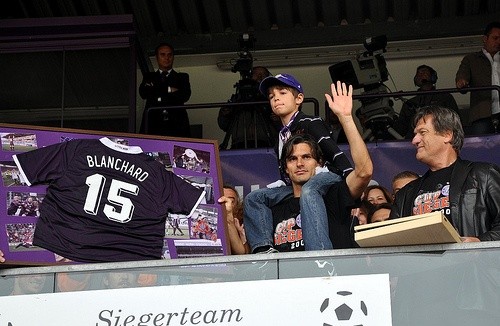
[259,73,303,97]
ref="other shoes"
[312,259,338,276]
[252,248,279,270]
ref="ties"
[162,70,168,83]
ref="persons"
[242,73,351,256]
[456,21,500,136]
[0,134,218,247]
[217,66,282,149]
[138,43,192,138]
[0,251,5,264]
[390,104,500,242]
[217,170,420,256]
[393,65,460,143]
[218,80,372,258]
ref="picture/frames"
[0,122,231,266]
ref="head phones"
[430,66,438,82]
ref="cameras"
[231,48,252,78]
